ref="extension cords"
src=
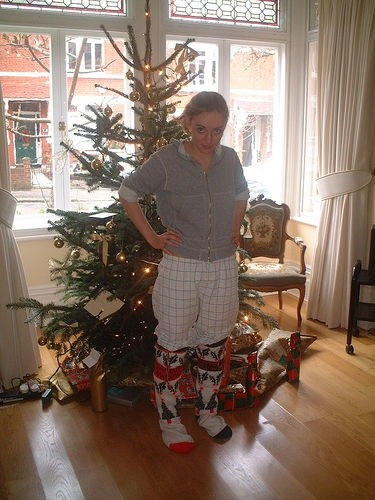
[0,389,46,400]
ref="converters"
[42,388,52,409]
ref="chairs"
[237,194,307,328]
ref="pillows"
[230,328,317,394]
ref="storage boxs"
[61,324,302,412]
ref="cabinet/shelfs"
[346,260,375,354]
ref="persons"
[117,90,249,455]
